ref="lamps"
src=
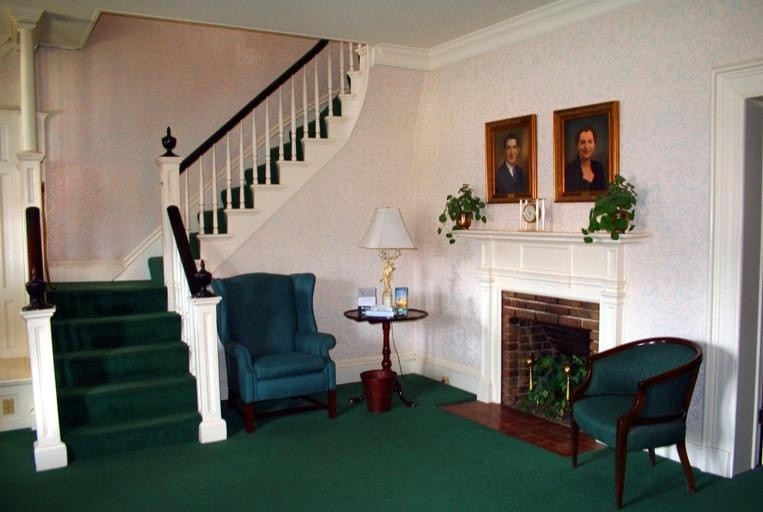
[355,203,417,306]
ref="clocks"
[516,198,546,233]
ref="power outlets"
[440,375,449,384]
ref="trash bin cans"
[360,370,396,412]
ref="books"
[364,309,394,317]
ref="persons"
[493,134,526,192]
[565,125,606,192]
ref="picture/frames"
[481,114,539,205]
[552,100,624,205]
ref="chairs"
[214,269,337,435]
[566,337,701,510]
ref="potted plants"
[436,185,488,243]
[583,172,637,242]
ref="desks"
[342,307,429,409]
